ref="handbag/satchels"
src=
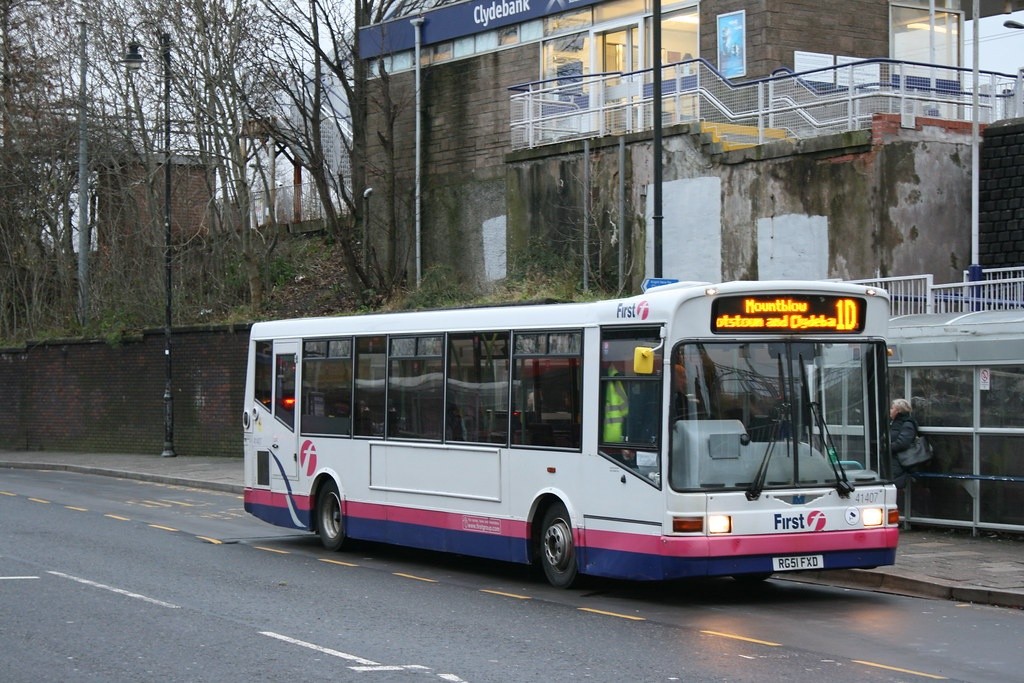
[896,419,935,469]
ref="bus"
[243,275,899,590]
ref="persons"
[623,365,690,460]
[889,399,917,489]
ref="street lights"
[116,19,176,456]
